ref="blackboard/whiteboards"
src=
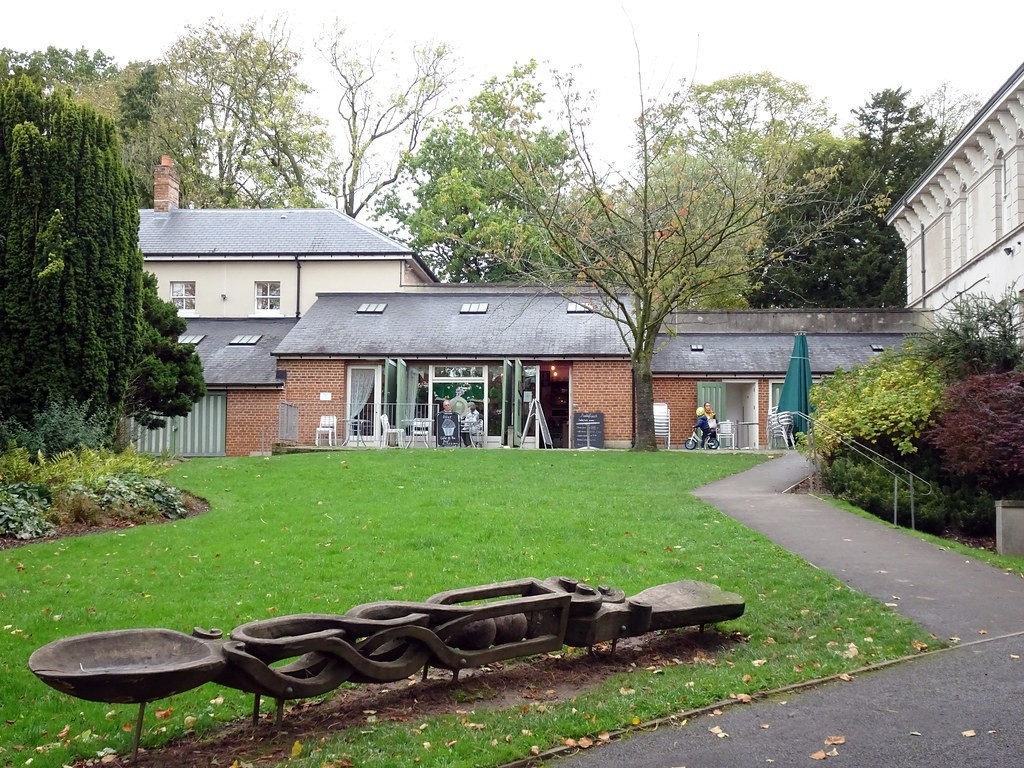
[574,412,604,449]
[436,411,460,448]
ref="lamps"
[1004,248,1013,255]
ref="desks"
[738,421,761,450]
[576,422,601,450]
[401,419,435,449]
[339,419,369,447]
[460,420,480,448]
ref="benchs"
[553,423,568,448]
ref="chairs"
[315,416,338,447]
[379,414,407,448]
[413,418,429,447]
[767,405,796,451]
[653,403,672,450]
[471,418,483,443]
[715,422,736,451]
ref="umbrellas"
[775,331,813,435]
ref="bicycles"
[684,426,720,450]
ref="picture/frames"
[541,371,551,387]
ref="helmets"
[696,407,705,416]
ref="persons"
[440,401,453,411]
[692,402,719,449]
[461,402,479,447]
